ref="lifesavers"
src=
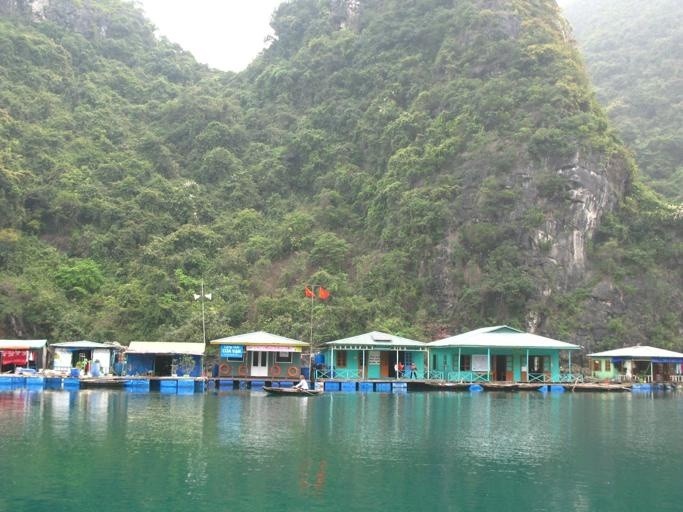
[270,365,281,376]
[218,363,231,376]
[237,364,249,378]
[286,366,299,378]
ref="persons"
[399,361,404,371]
[394,362,403,379]
[295,375,308,390]
[409,362,418,379]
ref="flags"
[319,287,329,299]
[305,288,315,298]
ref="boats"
[423,379,632,392]
[260,385,323,396]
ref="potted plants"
[178,354,196,378]
